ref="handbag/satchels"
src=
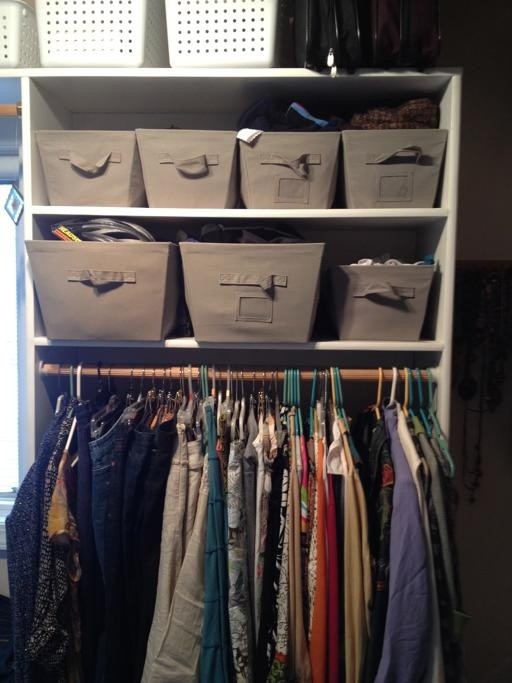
[291,0,442,71]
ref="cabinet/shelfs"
[1,68,464,682]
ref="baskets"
[0,1,277,68]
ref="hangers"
[33,358,458,486]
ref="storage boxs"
[237,131,341,208]
[34,128,148,208]
[342,129,448,208]
[25,239,180,340]
[179,241,327,345]
[328,263,434,341]
[134,128,242,209]
